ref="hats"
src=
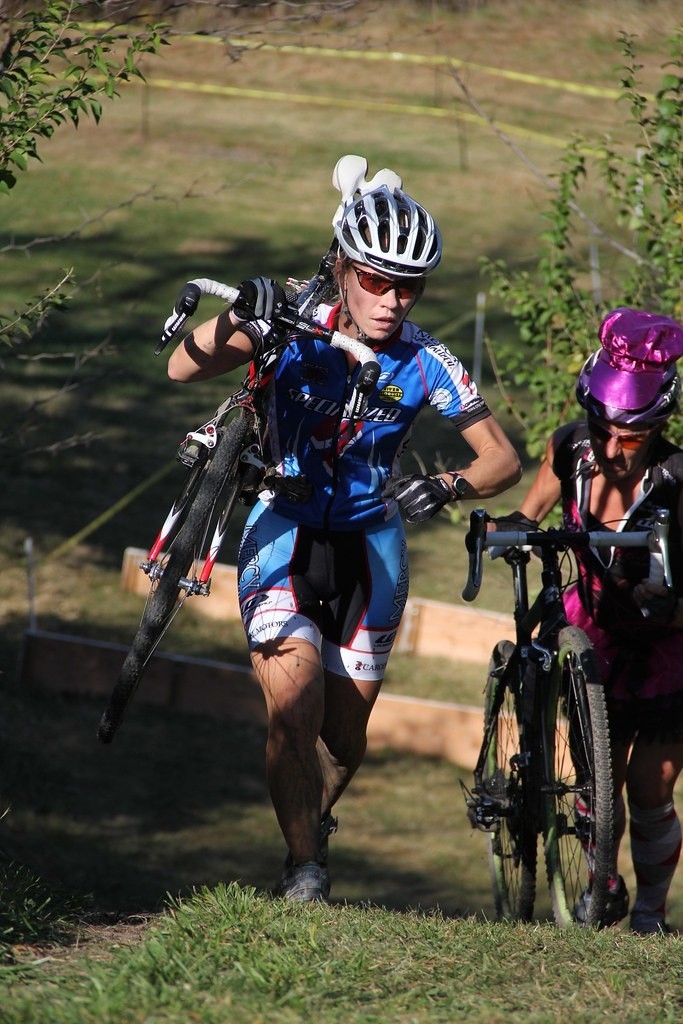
[589,305,683,410]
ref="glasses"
[588,417,664,451]
[346,262,424,299]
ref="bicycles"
[456,508,675,934]
[95,153,406,748]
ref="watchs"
[446,471,471,501]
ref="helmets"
[335,184,442,278]
[575,348,680,423]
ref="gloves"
[381,472,452,524]
[232,275,287,321]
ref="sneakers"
[283,809,338,869]
[282,862,330,902]
[631,909,668,934]
[571,875,630,931]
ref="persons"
[483,305,682,939]
[166,186,521,912]
